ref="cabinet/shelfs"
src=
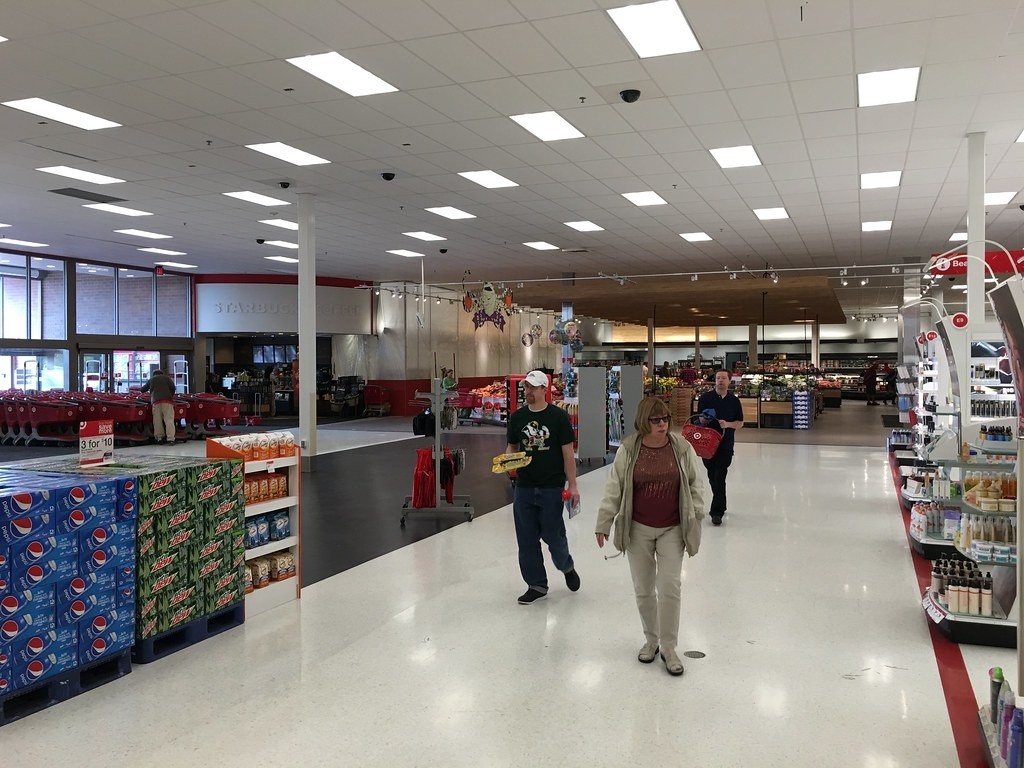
[207,431,299,621]
[885,361,1016,649]
[733,359,895,399]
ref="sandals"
[638,645,660,664]
[660,649,684,676]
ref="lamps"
[850,312,877,322]
[575,317,582,325]
[883,317,889,322]
[840,278,870,287]
[536,312,540,318]
[415,294,427,302]
[770,270,779,283]
[391,290,403,299]
[373,288,379,295]
[437,297,441,305]
[592,321,597,326]
[893,317,898,322]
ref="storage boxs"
[793,391,812,431]
[672,387,691,427]
[0,454,246,695]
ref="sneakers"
[518,588,547,605]
[564,569,581,591]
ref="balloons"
[549,316,584,353]
[522,324,542,347]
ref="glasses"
[648,415,672,424]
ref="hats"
[519,370,549,388]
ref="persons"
[507,371,581,605]
[140,369,176,446]
[595,397,704,675]
[861,362,898,406]
[643,361,648,377]
[705,363,721,382]
[678,362,697,385]
[808,363,818,373]
[699,369,745,525]
[443,369,457,390]
[659,361,669,378]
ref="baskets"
[683,414,725,460]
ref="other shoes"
[158,440,164,445]
[168,441,176,446]
[712,516,723,525]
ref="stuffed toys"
[460,282,513,332]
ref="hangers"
[557,401,577,415]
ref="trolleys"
[0,388,241,446]
[448,389,483,427]
[244,392,262,426]
[361,385,392,417]
